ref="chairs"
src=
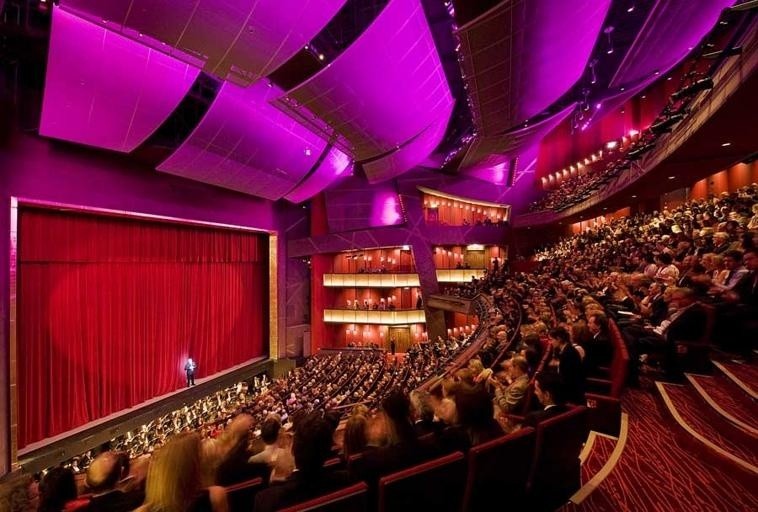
[226,403,586,512]
[673,300,715,354]
[584,318,630,430]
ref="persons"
[562,135,648,179]
[25,178,756,509]
